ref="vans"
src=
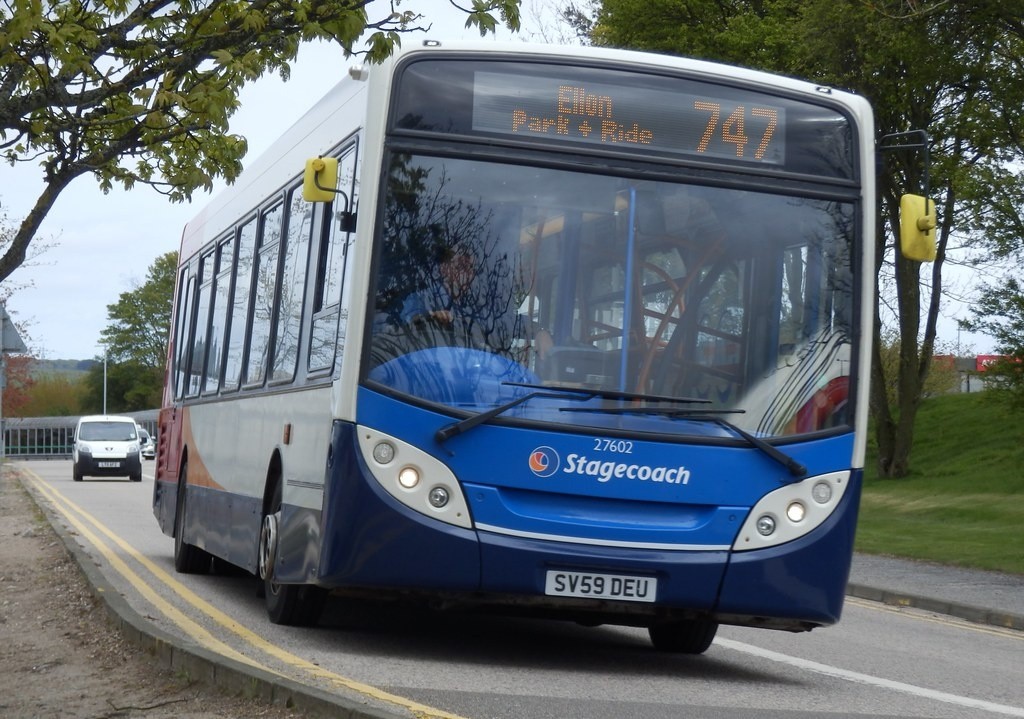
[70,415,142,482]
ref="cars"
[137,425,155,460]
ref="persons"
[401,236,554,385]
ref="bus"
[152,39,938,654]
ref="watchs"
[535,327,550,332]
